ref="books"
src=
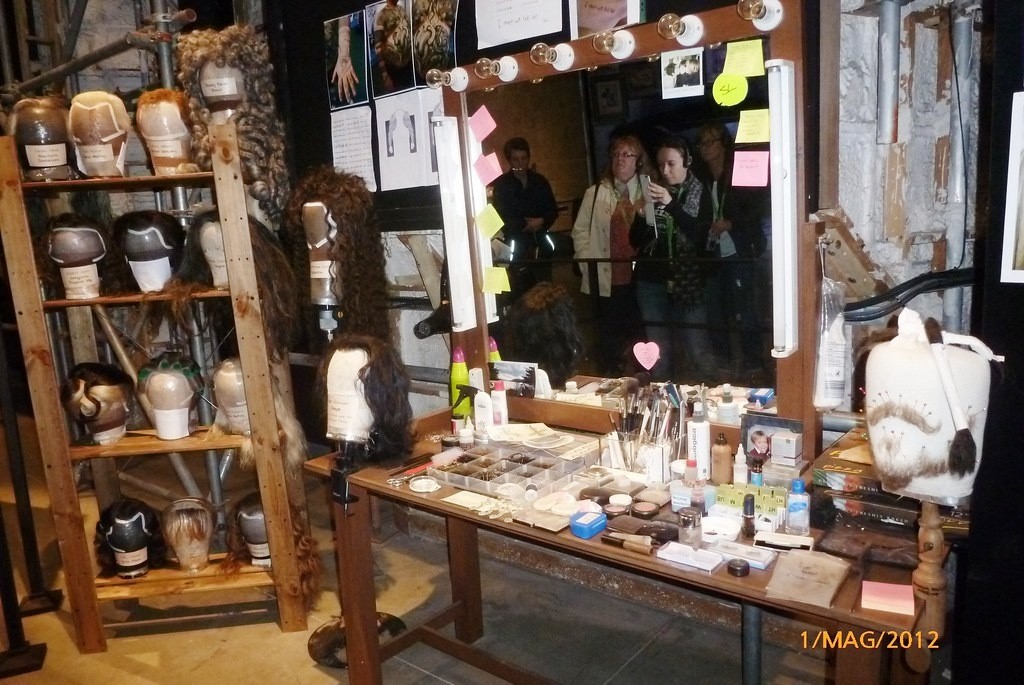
[860,580,914,614]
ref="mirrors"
[441,0,817,465]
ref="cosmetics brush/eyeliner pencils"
[608,385,676,447]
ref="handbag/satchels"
[665,254,705,295]
[574,282,594,319]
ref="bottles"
[459,429,475,450]
[451,347,470,413]
[466,416,474,429]
[441,436,460,451]
[474,428,488,446]
[784,479,811,536]
[451,414,465,436]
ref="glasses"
[696,138,721,148]
[610,151,638,159]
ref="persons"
[664,60,700,89]
[569,121,768,383]
[331,17,359,102]
[492,138,559,293]
[747,430,772,467]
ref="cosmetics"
[579,400,810,576]
[684,382,773,426]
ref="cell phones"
[640,174,653,201]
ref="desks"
[303,413,949,685]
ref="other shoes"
[748,366,769,388]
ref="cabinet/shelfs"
[0,122,306,652]
